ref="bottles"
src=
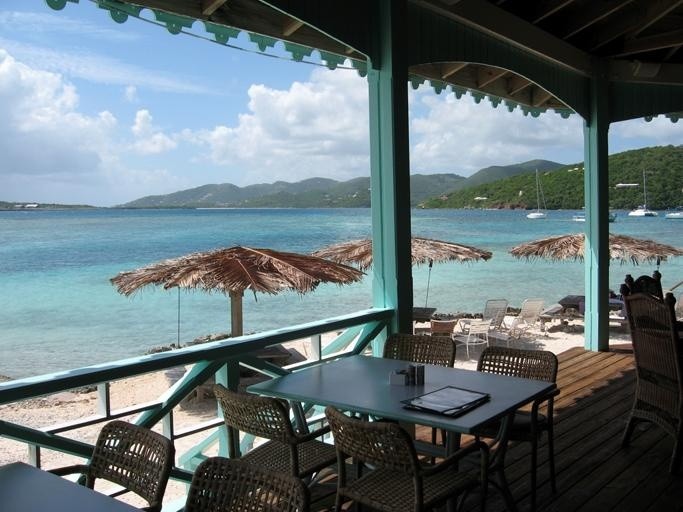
[417,363,425,385]
[409,364,416,385]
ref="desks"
[0,462,148,512]
[251,353,555,512]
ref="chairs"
[476,347,560,512]
[430,297,545,360]
[382,333,456,465]
[213,382,352,512]
[47,421,174,512]
[184,456,311,512]
[618,284,682,473]
[624,270,663,298]
[324,405,490,512]
